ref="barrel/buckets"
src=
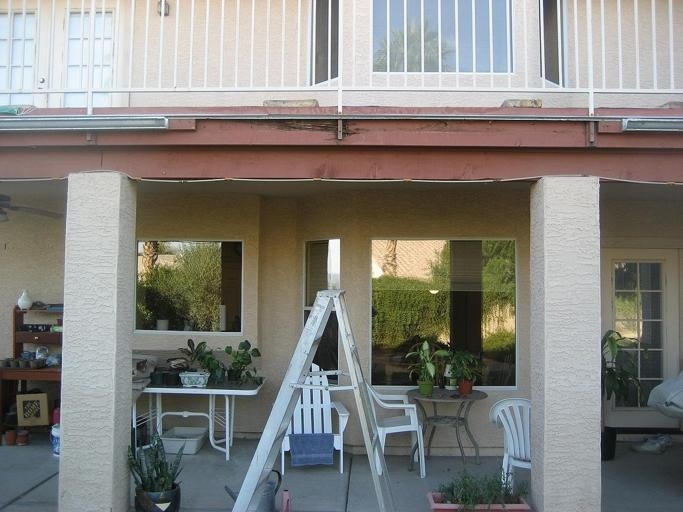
[51,424,60,456]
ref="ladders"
[232,290,392,512]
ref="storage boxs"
[17,387,57,428]
[161,427,209,455]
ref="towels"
[287,433,333,469]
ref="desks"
[406,387,489,470]
[130,372,265,461]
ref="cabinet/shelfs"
[1,303,64,430]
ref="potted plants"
[427,462,532,512]
[128,426,190,512]
[601,331,647,461]
[449,350,480,394]
[175,337,215,388]
[405,340,449,396]
[225,339,262,381]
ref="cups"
[4,429,30,445]
[0,358,47,370]
[164,371,178,386]
[150,373,163,385]
[156,320,170,330]
[23,323,46,332]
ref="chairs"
[361,375,428,479]
[489,396,532,484]
[281,362,349,475]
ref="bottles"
[281,488,292,512]
[17,290,33,310]
[438,371,445,389]
[53,407,60,424]
